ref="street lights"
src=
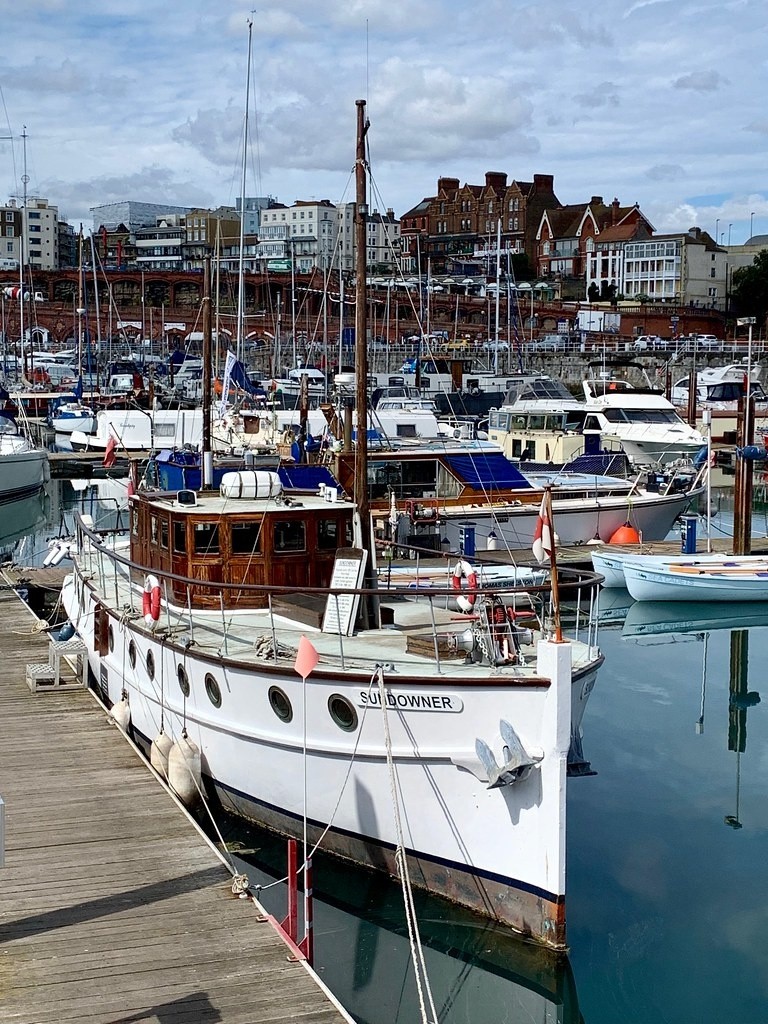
[723,631,744,832]
[735,316,759,556]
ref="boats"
[589,587,638,628]
[55,98,610,956]
[619,600,768,648]
[188,796,588,1024]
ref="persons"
[588,282,599,302]
[607,281,619,301]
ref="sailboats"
[0,9,768,621]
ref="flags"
[532,492,558,565]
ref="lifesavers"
[452,560,477,612]
[127,462,138,495]
[143,574,161,630]
